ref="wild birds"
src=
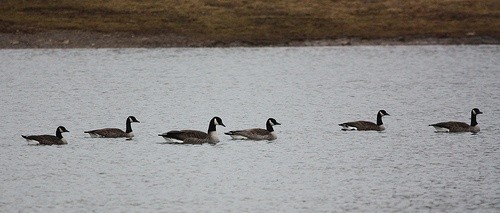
[84,116,140,138]
[429,108,484,133]
[158,117,226,144]
[338,110,390,131]
[21,126,69,145]
[224,118,281,140]
